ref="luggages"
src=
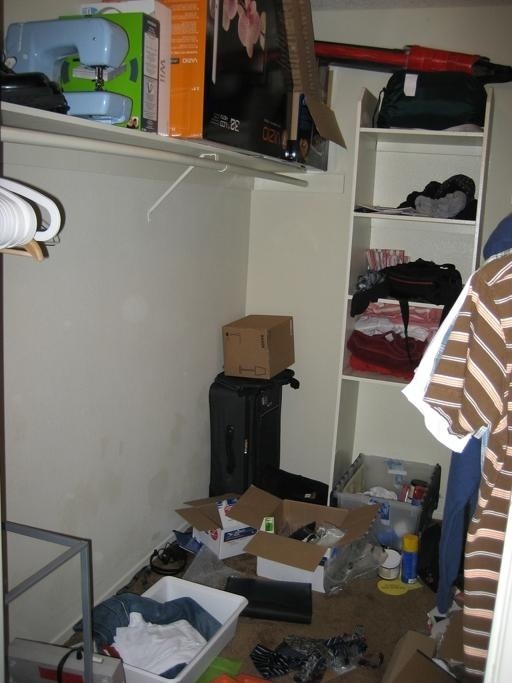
[209,380,282,500]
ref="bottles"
[401,534,419,584]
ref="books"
[224,575,313,624]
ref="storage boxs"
[102,574,249,683]
[179,451,444,596]
[220,313,295,380]
[60,0,350,171]
[383,631,439,683]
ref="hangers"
[1,176,64,263]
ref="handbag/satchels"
[376,69,487,129]
[380,257,463,305]
[215,368,301,400]
[345,330,427,380]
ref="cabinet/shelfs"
[324,90,500,509]
